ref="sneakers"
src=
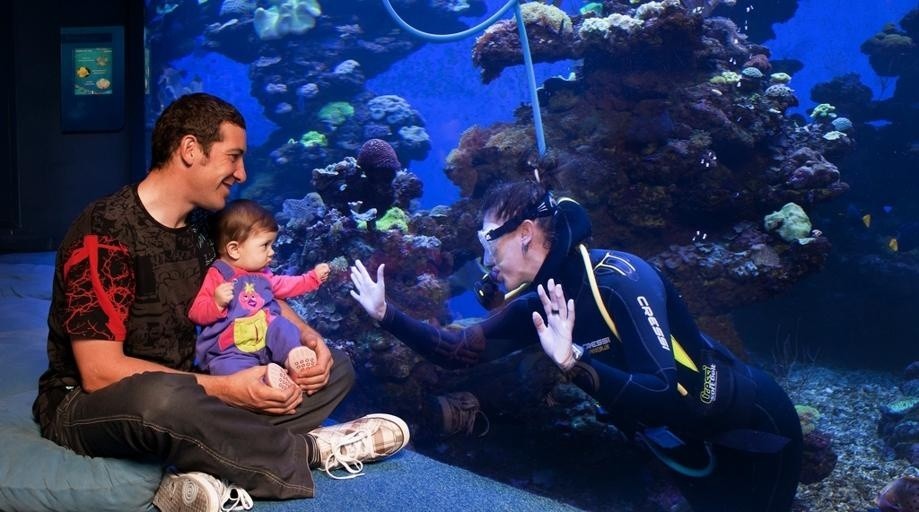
[152,470,234,512]
[303,412,412,473]
[283,345,319,394]
[265,361,304,405]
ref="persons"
[344,179,802,510]
[31,90,410,512]
[187,198,330,405]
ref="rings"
[551,310,558,315]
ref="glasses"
[476,189,557,257]
